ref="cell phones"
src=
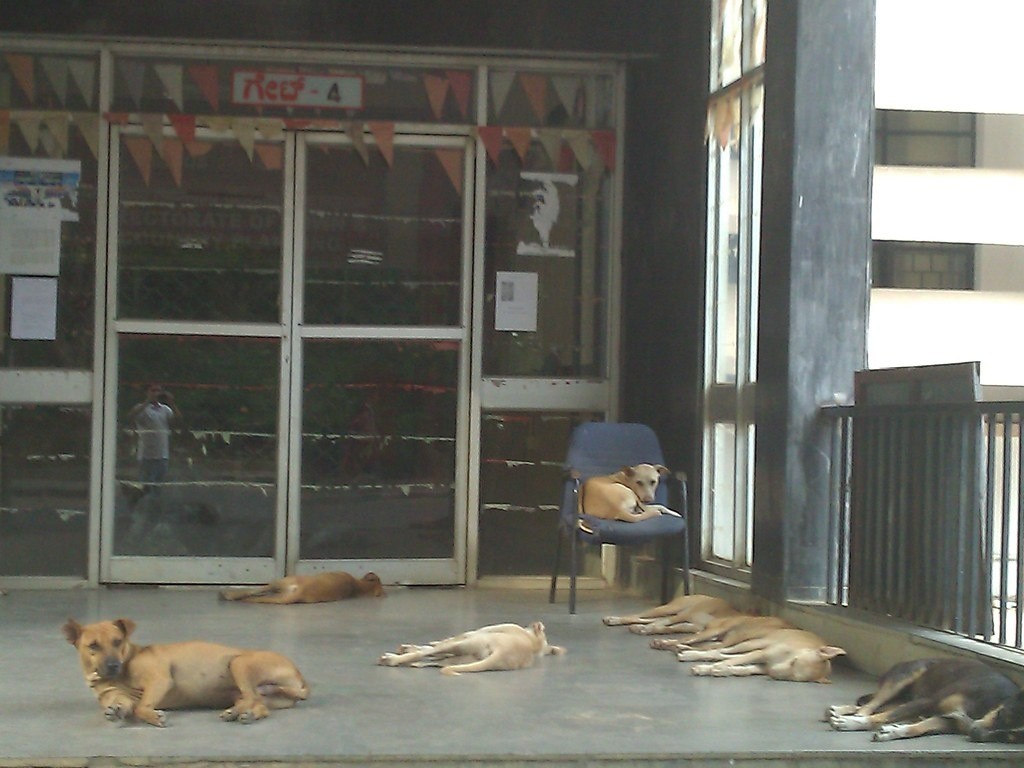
[156,395,166,401]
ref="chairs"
[548,421,691,585]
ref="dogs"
[376,621,564,677]
[601,594,847,684]
[824,653,1023,743]
[60,617,310,729]
[218,571,386,605]
[577,464,684,523]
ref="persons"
[119,383,185,522]
